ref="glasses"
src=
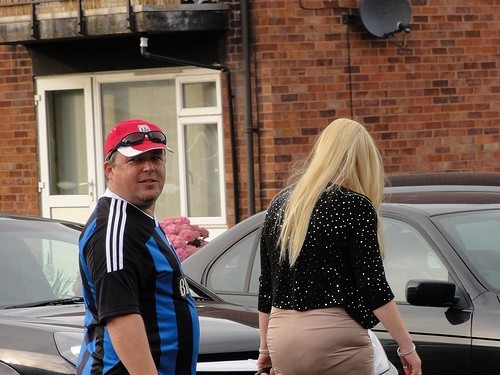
[108,130,166,159]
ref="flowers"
[159,216,210,262]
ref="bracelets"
[397,343,416,355]
[259,349,269,354]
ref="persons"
[256,118,422,375]
[76,118,200,374]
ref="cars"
[0,214,398,375]
[180,173,500,375]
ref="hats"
[104,118,174,158]
